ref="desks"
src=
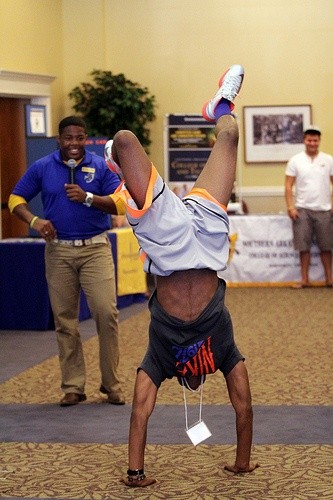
[0,214,328,332]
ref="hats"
[303,126,321,135]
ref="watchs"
[83,191,93,207]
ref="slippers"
[291,282,312,289]
[326,284,333,288]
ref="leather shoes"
[100,386,125,405]
[60,393,86,405]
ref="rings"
[45,231,49,235]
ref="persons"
[104,64,261,488]
[285,125,333,289]
[9,116,122,405]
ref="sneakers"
[104,140,125,182]
[202,64,244,121]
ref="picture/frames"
[242,104,312,165]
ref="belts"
[52,231,107,247]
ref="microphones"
[68,158,77,184]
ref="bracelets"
[29,216,38,229]
[127,469,146,482]
